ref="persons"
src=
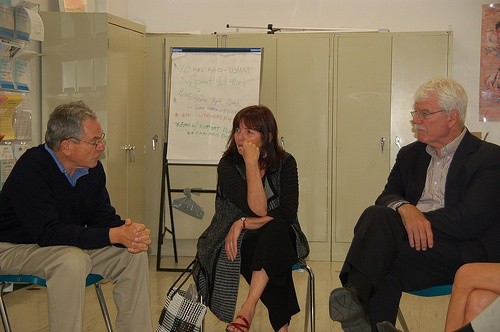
[329,77,500,332]
[0,99,152,332]
[192,105,310,332]
[443,262,500,332]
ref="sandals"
[225,314,250,332]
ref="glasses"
[410,110,444,120]
[61,132,106,151]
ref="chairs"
[0,274,115,332]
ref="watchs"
[241,217,246,229]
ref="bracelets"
[396,203,411,214]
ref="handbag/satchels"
[156,259,208,332]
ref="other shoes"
[329,287,371,331]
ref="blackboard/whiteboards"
[166,47,261,166]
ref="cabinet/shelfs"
[147,32,454,262]
[39,10,147,226]
